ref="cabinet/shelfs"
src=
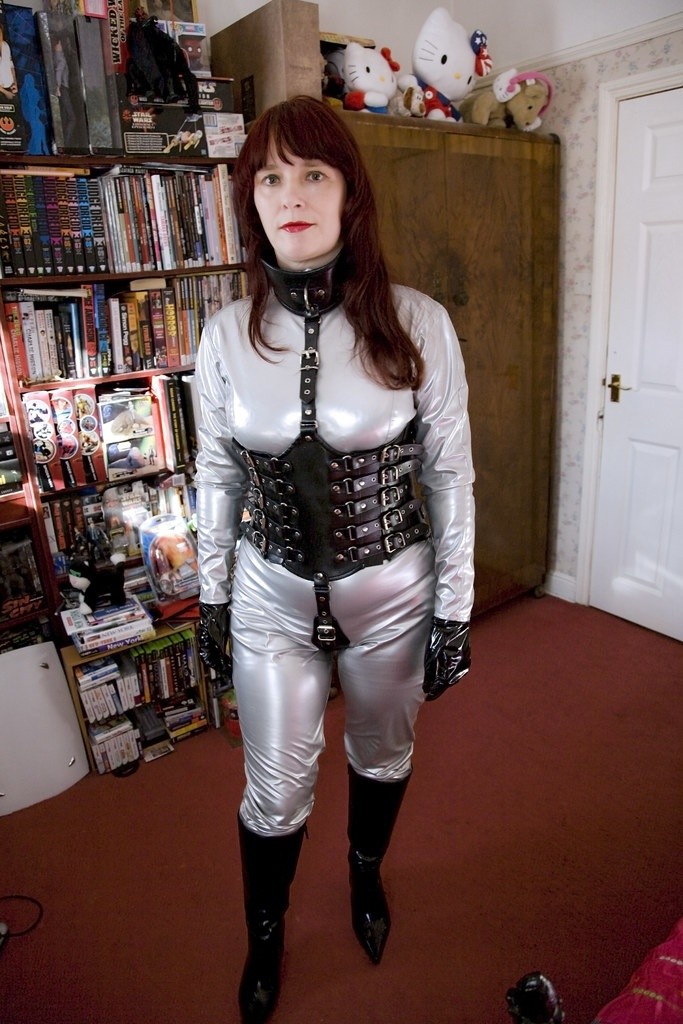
[0,346,61,653]
[322,109,562,673]
[59,619,211,775]
[0,155,257,643]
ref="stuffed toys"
[69,551,129,615]
[318,6,559,134]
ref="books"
[0,161,264,786]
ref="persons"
[187,94,483,1024]
[0,22,20,141]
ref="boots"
[346,760,412,966]
[236,814,307,1024]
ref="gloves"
[197,600,234,676]
[421,616,471,701]
